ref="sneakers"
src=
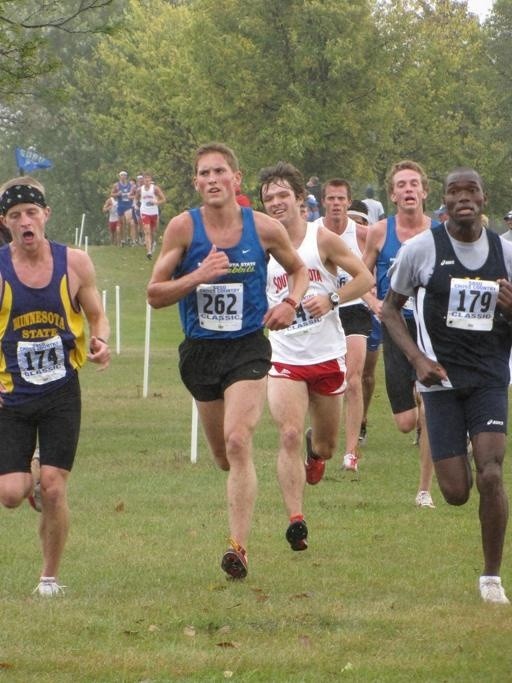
[416,489,435,509]
[304,428,325,485]
[29,447,44,512]
[341,453,359,473]
[286,514,308,551]
[478,574,511,606]
[32,577,67,598]
[221,538,249,578]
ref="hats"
[433,204,447,215]
[504,211,512,220]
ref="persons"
[0,176,110,596]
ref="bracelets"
[89,337,107,354]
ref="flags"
[14,146,52,172]
